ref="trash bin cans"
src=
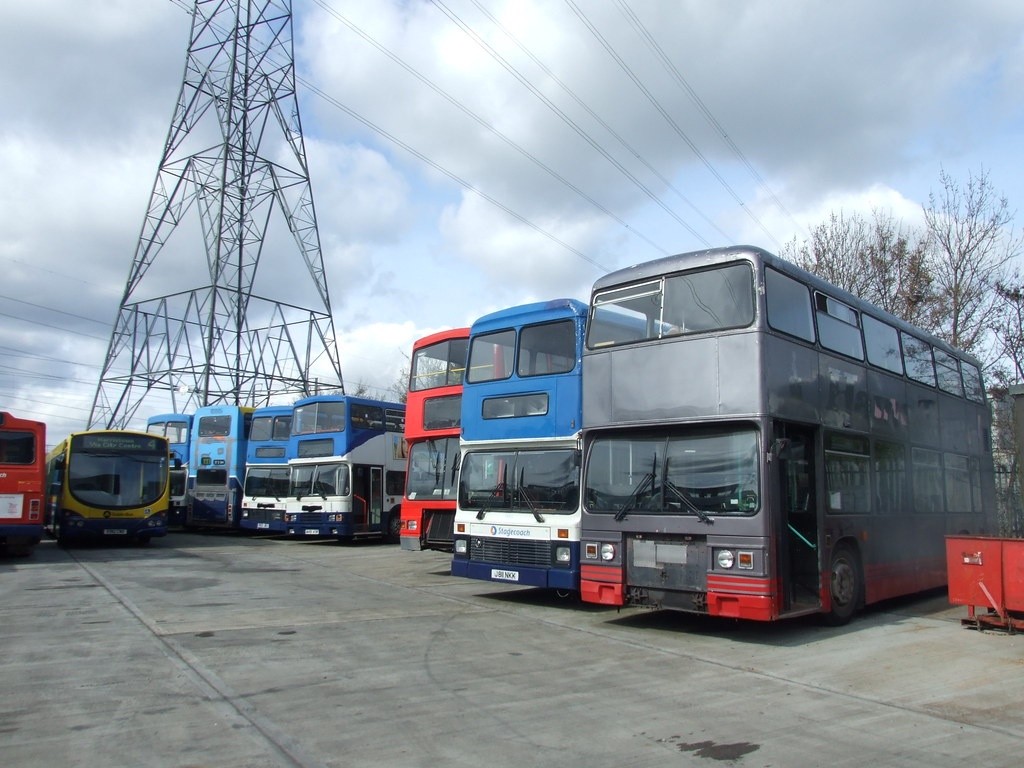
[943,535,1024,630]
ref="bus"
[284,395,445,544]
[1,412,47,557]
[239,406,404,532]
[579,245,999,626]
[452,299,670,608]
[145,414,198,532]
[186,405,255,537]
[399,328,575,553]
[45,430,182,547]
